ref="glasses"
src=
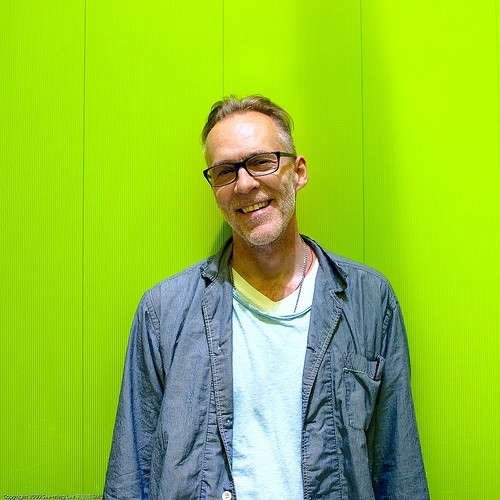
[203,151,297,188]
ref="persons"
[102,94,430,500]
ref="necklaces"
[230,236,307,314]
